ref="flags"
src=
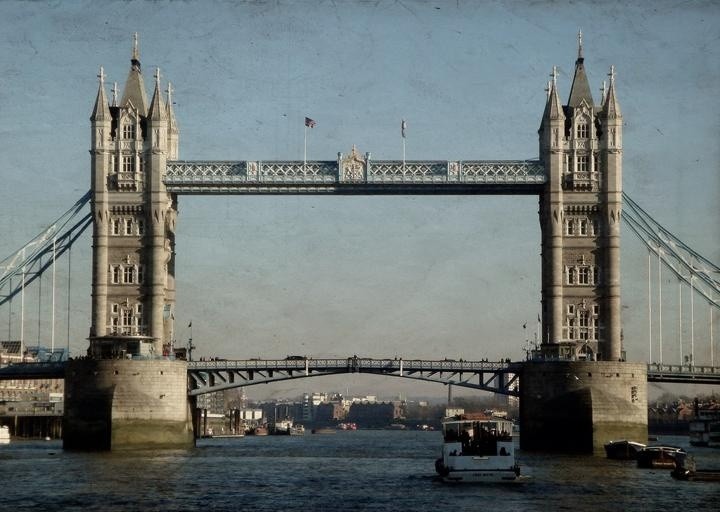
[304,117,317,128]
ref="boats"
[603,437,719,482]
[435,410,522,484]
